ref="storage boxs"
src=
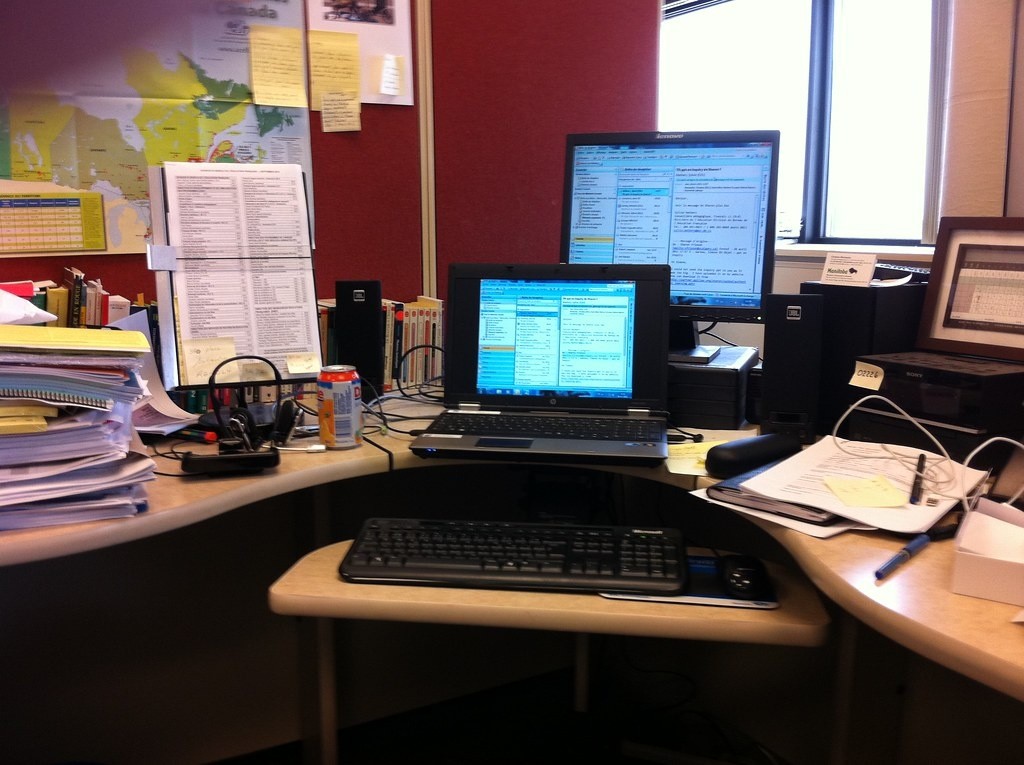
[950,500,1023,609]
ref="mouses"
[715,554,776,601]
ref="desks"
[0,383,1023,765]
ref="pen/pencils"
[909,453,928,503]
[877,531,934,581]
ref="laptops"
[856,217,1024,387]
[408,261,671,466]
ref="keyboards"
[341,516,703,596]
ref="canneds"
[318,365,363,449]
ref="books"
[669,344,760,373]
[0,267,316,414]
[316,295,443,392]
[0,324,158,531]
[705,457,845,527]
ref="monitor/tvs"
[559,130,780,363]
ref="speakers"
[760,293,824,443]
[334,279,384,404]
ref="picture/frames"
[915,216,1024,363]
